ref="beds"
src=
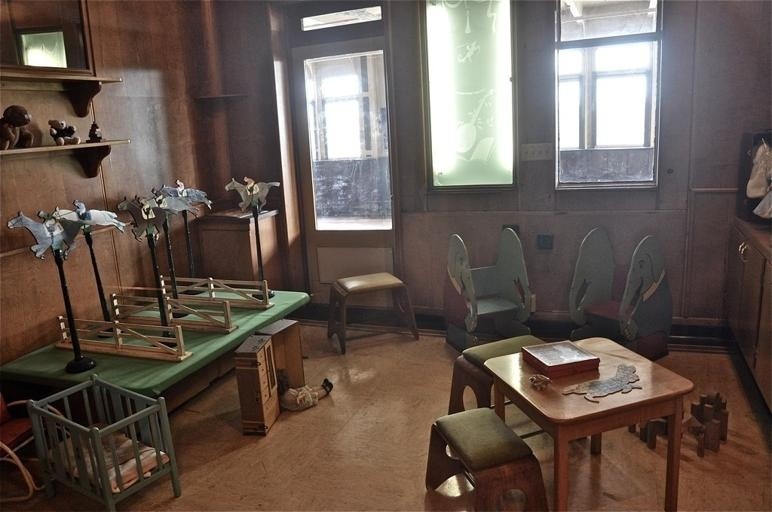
[26,372,182,512]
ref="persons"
[277,369,333,413]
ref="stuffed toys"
[47,118,81,146]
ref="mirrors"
[1,1,92,81]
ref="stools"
[446,334,549,415]
[424,406,551,512]
[325,270,420,355]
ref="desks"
[480,335,697,512]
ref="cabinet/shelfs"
[0,69,139,257]
[725,217,772,418]
[189,205,282,291]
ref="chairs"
[569,226,671,361]
[443,227,531,351]
[0,389,74,504]
